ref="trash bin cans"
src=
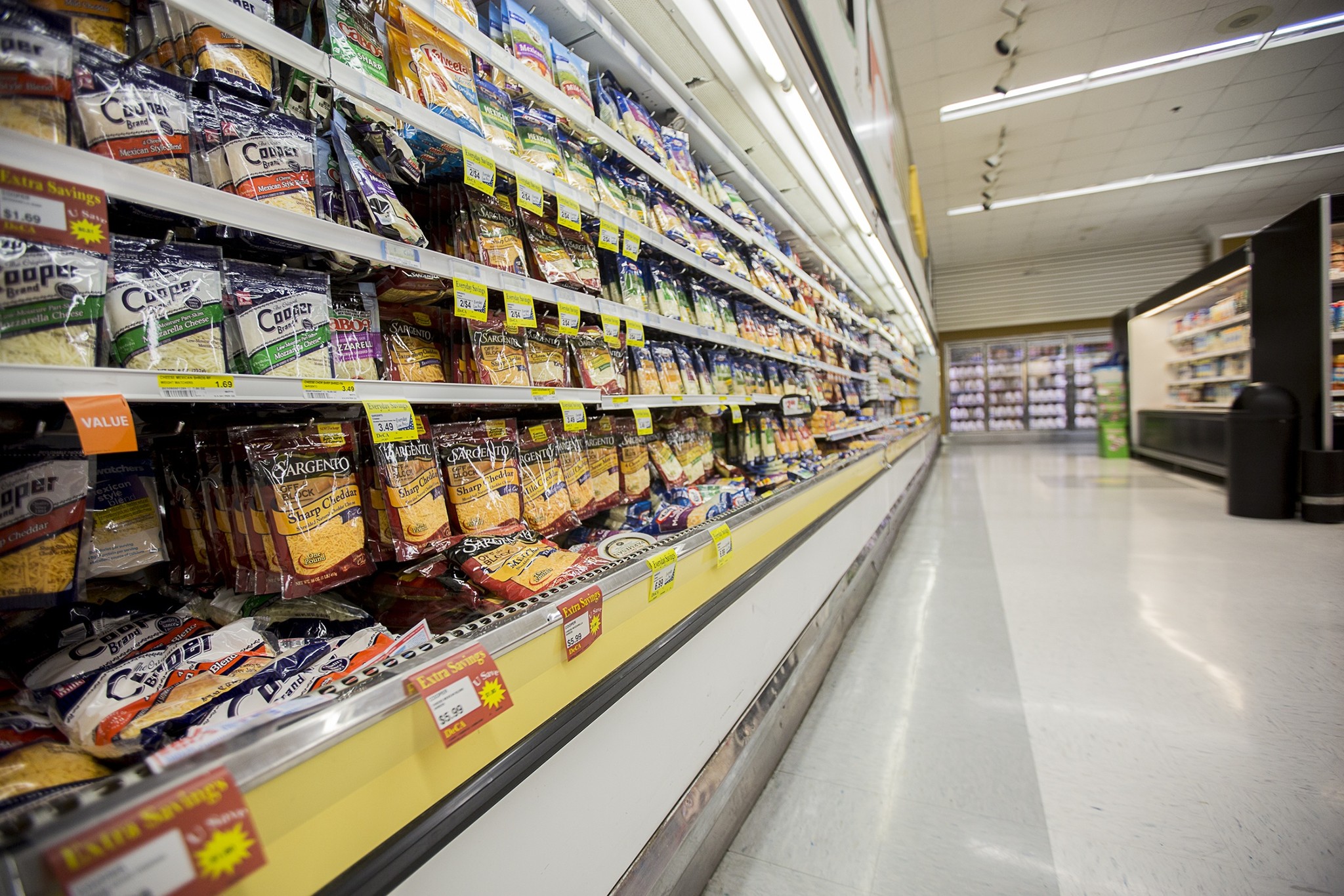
[1227,381,1298,521]
[1297,448,1344,524]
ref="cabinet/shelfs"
[1,1,1344,895]
